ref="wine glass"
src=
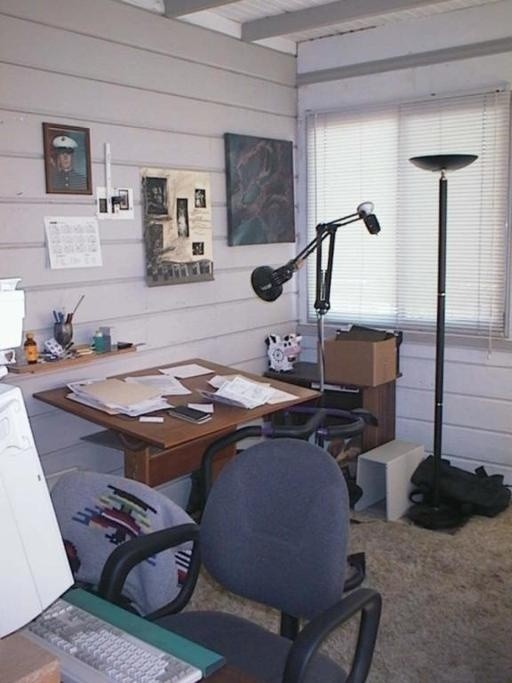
[53,321,74,360]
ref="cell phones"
[172,405,212,422]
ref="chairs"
[99,438,382,681]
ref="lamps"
[250,201,380,407]
[406,152,481,531]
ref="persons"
[50,135,86,188]
[176,199,189,237]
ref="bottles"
[24,332,38,365]
[93,329,104,354]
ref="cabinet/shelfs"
[261,360,397,460]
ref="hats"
[52,136,79,154]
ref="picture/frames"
[41,121,94,196]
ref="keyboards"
[15,596,202,683]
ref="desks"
[31,357,322,516]
[22,587,229,682]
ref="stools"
[353,438,425,523]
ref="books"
[166,405,212,425]
[63,377,173,416]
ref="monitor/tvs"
[0,382,75,642]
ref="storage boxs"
[316,329,399,387]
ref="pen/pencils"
[53,296,84,323]
